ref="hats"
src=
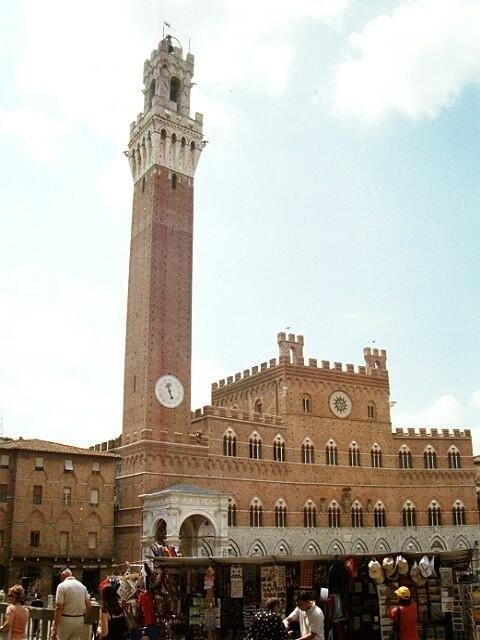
[395,586,411,599]
[344,558,358,578]
[368,554,408,584]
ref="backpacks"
[410,555,437,586]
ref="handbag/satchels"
[1,629,12,639]
[388,606,400,640]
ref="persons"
[97,586,129,640]
[389,586,420,640]
[31,594,44,637]
[51,565,92,640]
[0,584,30,640]
[247,597,294,640]
[282,591,325,640]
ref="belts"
[61,614,85,617]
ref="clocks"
[153,372,186,409]
